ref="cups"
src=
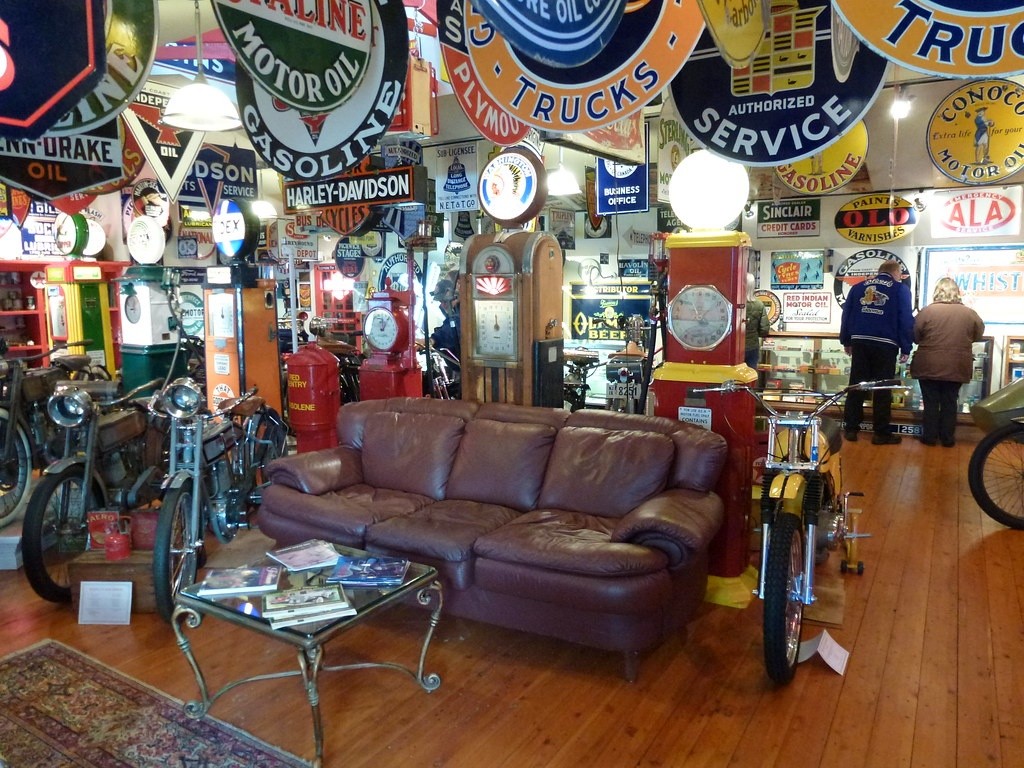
[13,299,23,309]
[8,291,18,299]
[2,299,12,309]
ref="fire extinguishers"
[104,516,133,560]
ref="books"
[261,582,358,631]
[198,564,283,595]
[265,538,342,571]
[326,555,411,588]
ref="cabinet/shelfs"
[755,330,994,425]
[1006,334,1024,386]
[101,261,134,371]
[0,259,50,368]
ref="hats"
[433,280,453,300]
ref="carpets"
[0,637,310,768]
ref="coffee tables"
[167,542,445,768]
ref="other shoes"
[871,433,902,444]
[919,435,937,446]
[943,442,955,447]
[844,428,857,441]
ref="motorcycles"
[281,328,462,438]
[692,378,914,686]
[968,417,1024,531]
[0,339,123,528]
[147,377,288,625]
[21,376,211,604]
[593,313,651,414]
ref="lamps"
[252,168,278,217]
[888,83,912,119]
[157,0,243,133]
[666,149,754,248]
[546,145,584,198]
[582,265,617,298]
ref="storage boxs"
[782,396,799,402]
[1011,367,1024,382]
[130,508,183,550]
[69,550,157,615]
[757,364,772,369]
[971,342,988,354]
[1013,342,1020,354]
[1012,353,1024,361]
[1009,343,1014,360]
[87,506,126,552]
[763,390,782,401]
[765,378,783,389]
[804,398,816,404]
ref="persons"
[838,259,914,446]
[909,277,985,448]
[743,272,770,373]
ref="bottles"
[0,272,8,284]
[25,296,35,309]
[10,272,18,284]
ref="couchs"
[250,395,730,688]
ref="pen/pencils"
[305,568,324,583]
